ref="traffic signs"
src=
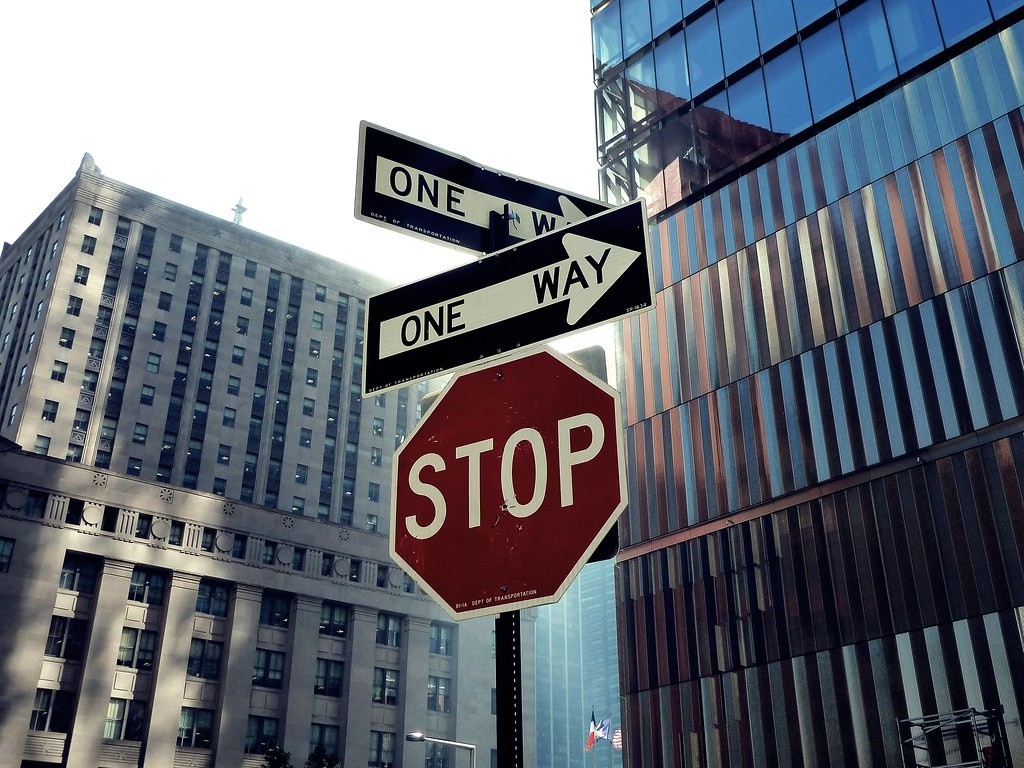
[360,197,658,401]
[353,115,621,260]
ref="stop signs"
[390,341,631,622]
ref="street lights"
[405,731,477,768]
